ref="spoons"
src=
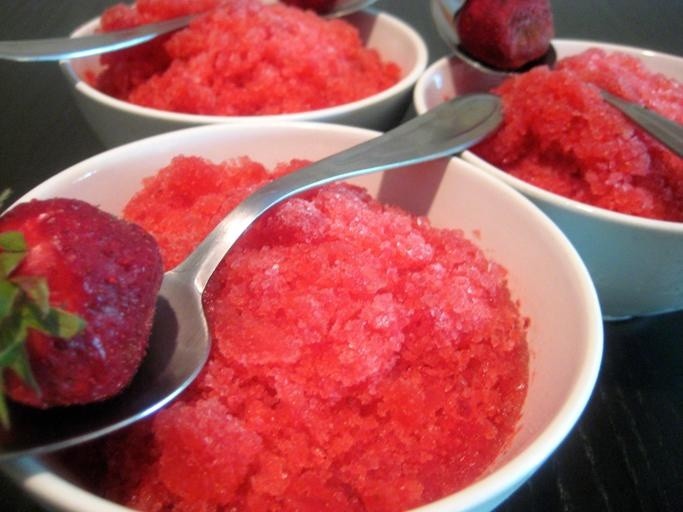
[429,0,683,159]
[3,83,509,459]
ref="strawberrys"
[0,197,164,409]
[455,0,554,69]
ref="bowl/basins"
[411,37,682,323]
[58,0,430,152]
[5,121,605,512]
